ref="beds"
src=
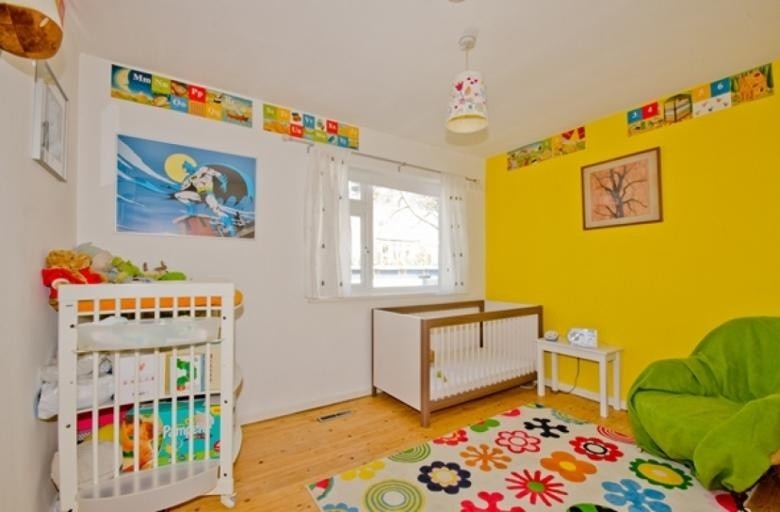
[369,298,543,430]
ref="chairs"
[627,315,780,492]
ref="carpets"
[306,401,758,511]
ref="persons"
[122,416,154,473]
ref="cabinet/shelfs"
[53,279,244,509]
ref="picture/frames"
[580,146,664,232]
[30,55,70,185]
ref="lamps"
[443,35,488,133]
